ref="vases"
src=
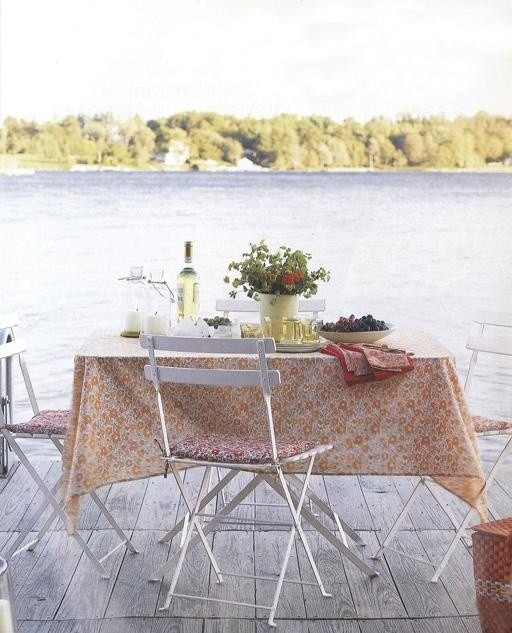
[256,293,300,324]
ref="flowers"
[223,238,332,307]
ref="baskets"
[471,518,512,632]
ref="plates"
[317,323,396,344]
[275,342,322,353]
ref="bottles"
[175,240,200,324]
[120,265,172,336]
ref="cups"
[279,316,313,345]
[239,324,264,339]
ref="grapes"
[320,314,388,333]
[203,316,230,329]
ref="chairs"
[372,308,511,584]
[137,330,334,629]
[1,313,138,583]
